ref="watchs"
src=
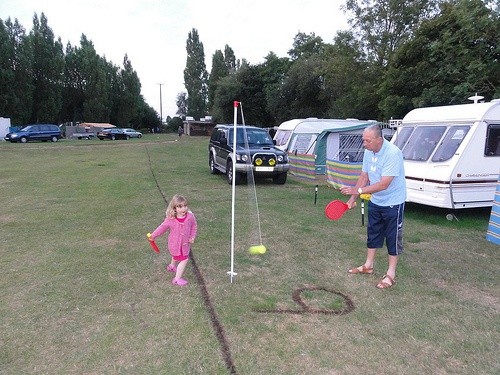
[357,187,363,195]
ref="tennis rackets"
[326,200,356,220]
[147,233,159,252]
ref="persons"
[339,124,407,289]
[149,195,197,286]
[178,126,183,138]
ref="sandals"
[377,274,396,289]
[349,265,374,275]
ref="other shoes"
[172,278,188,286]
[167,264,177,272]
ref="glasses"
[176,203,187,208]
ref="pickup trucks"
[73,129,95,139]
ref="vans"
[4,124,63,143]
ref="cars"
[97,129,130,140]
[121,129,142,138]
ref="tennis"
[249,245,265,254]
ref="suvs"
[207,124,290,186]
